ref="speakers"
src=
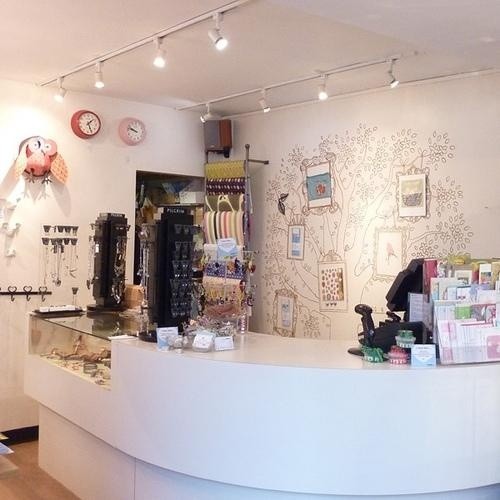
[204,120,232,150]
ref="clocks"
[71,109,148,145]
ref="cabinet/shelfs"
[133,143,268,338]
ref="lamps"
[198,59,398,125]
[51,13,230,104]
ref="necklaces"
[136,240,151,309]
[41,243,79,287]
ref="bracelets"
[83,362,98,375]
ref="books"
[37,304,84,314]
[423,255,500,364]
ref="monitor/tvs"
[386,258,425,322]
